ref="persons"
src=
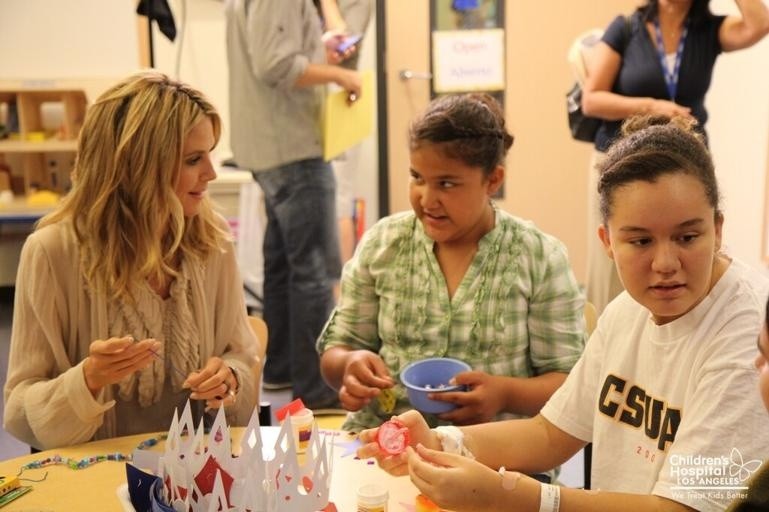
[726,303,768,510]
[3,71,261,452]
[314,94,589,484]
[580,0,769,170]
[224,1,366,411]
[355,115,769,510]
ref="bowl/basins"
[401,358,472,414]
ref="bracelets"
[433,423,465,453]
[541,482,562,509]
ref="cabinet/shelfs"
[0,87,90,300]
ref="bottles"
[274,399,314,465]
[355,484,389,512]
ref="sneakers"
[258,370,357,417]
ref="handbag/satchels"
[566,84,602,143]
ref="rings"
[222,380,232,389]
[230,391,237,402]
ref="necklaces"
[660,14,679,39]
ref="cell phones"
[333,33,365,54]
